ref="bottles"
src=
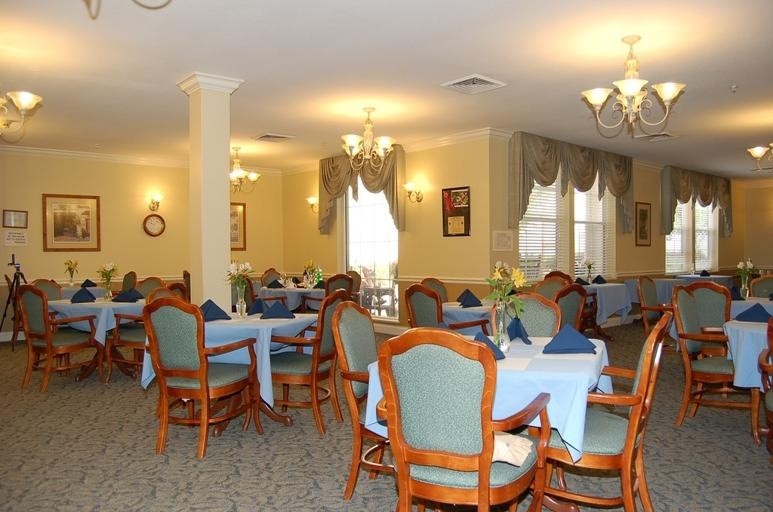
[314,270,319,285]
[318,269,323,283]
[303,270,308,283]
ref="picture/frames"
[42,194,101,251]
[635,202,651,246]
[3,209,28,229]
[491,230,514,251]
[443,186,470,237]
[230,203,247,251]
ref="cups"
[286,277,292,288]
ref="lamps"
[747,141,773,171]
[337,107,395,175]
[580,36,686,138]
[405,182,422,202]
[306,195,319,214]
[229,146,261,193]
[0,91,43,143]
[148,192,163,211]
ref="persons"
[454,192,461,205]
[461,191,469,205]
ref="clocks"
[143,214,165,236]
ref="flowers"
[580,258,598,274]
[224,258,255,300]
[304,259,323,283]
[484,261,528,319]
[96,262,119,285]
[737,258,754,288]
[63,256,80,274]
